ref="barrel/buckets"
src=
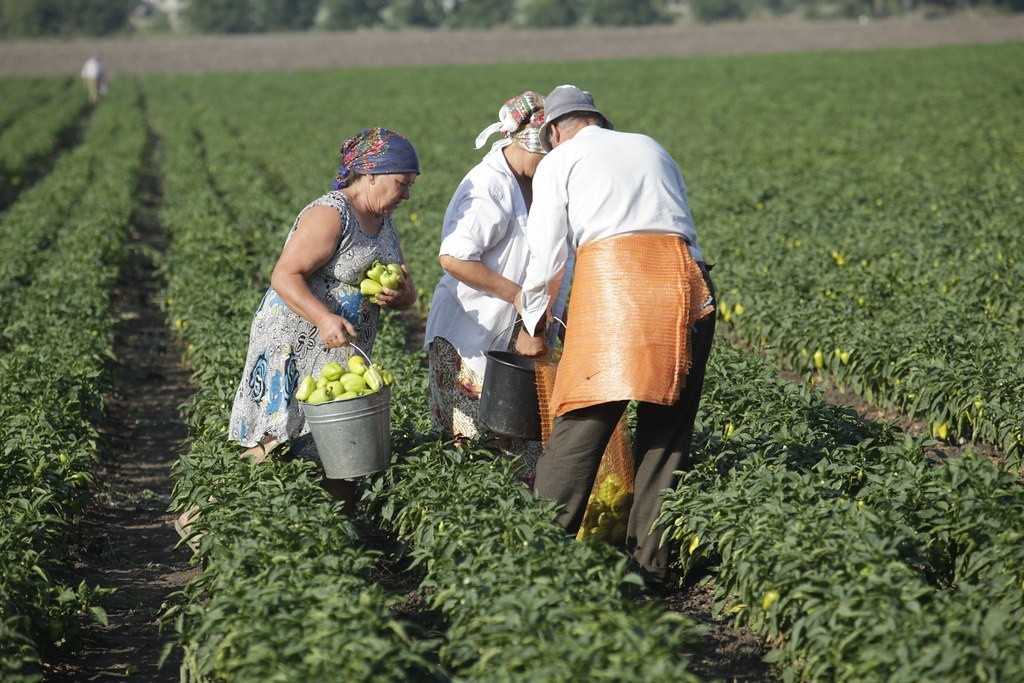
[297,340,391,479]
[476,311,567,439]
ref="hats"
[538,84,614,153]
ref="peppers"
[360,259,404,302]
[576,472,631,546]
[294,355,393,404]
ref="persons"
[226,126,420,461]
[426,84,717,592]
[82,54,104,104]
